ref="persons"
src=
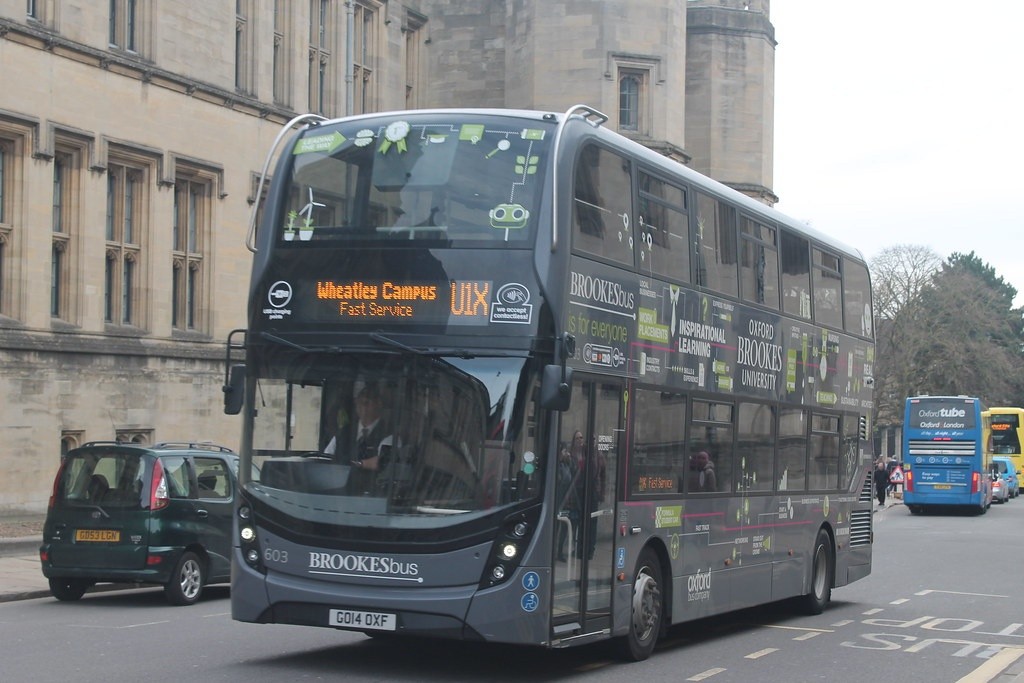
[560,430,606,584]
[876,454,884,463]
[887,455,898,496]
[874,463,891,505]
[197,470,225,497]
[690,452,716,492]
[325,387,401,472]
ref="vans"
[991,456,1022,498]
[39,440,262,606]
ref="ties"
[355,428,369,446]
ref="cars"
[991,472,1009,504]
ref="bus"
[902,395,997,515]
[989,407,1024,487]
[222,104,876,662]
